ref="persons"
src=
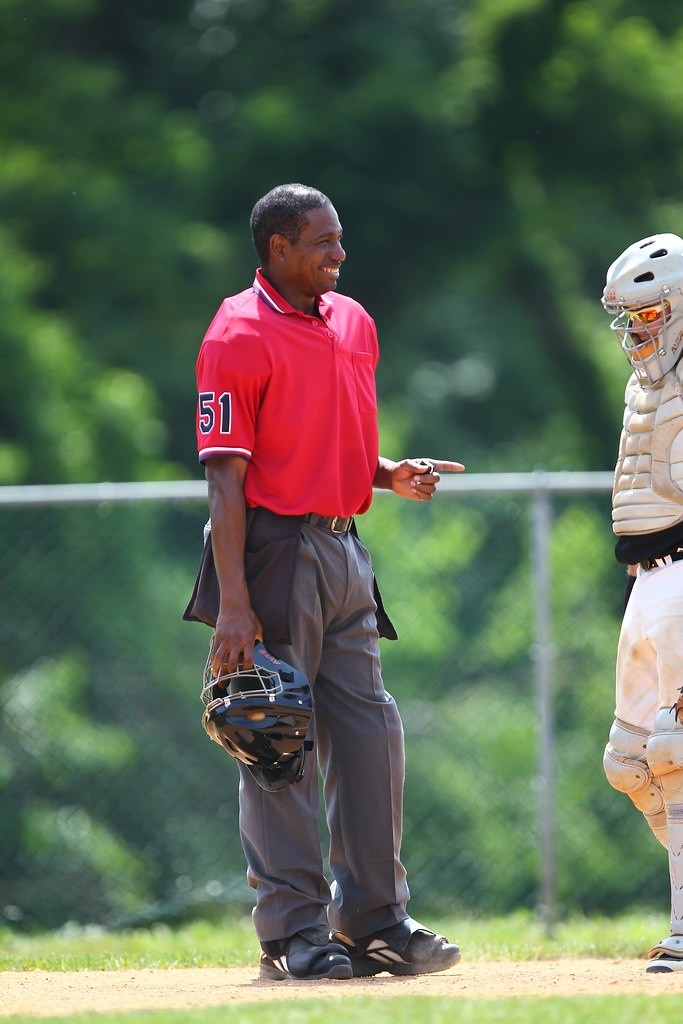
[599,233,682,975]
[194,176,469,985]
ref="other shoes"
[645,949,683,973]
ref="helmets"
[201,642,316,793]
[600,233,683,389]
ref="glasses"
[623,301,670,325]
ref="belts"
[283,508,356,536]
[639,547,683,571]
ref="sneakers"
[331,927,462,977]
[258,931,356,980]
